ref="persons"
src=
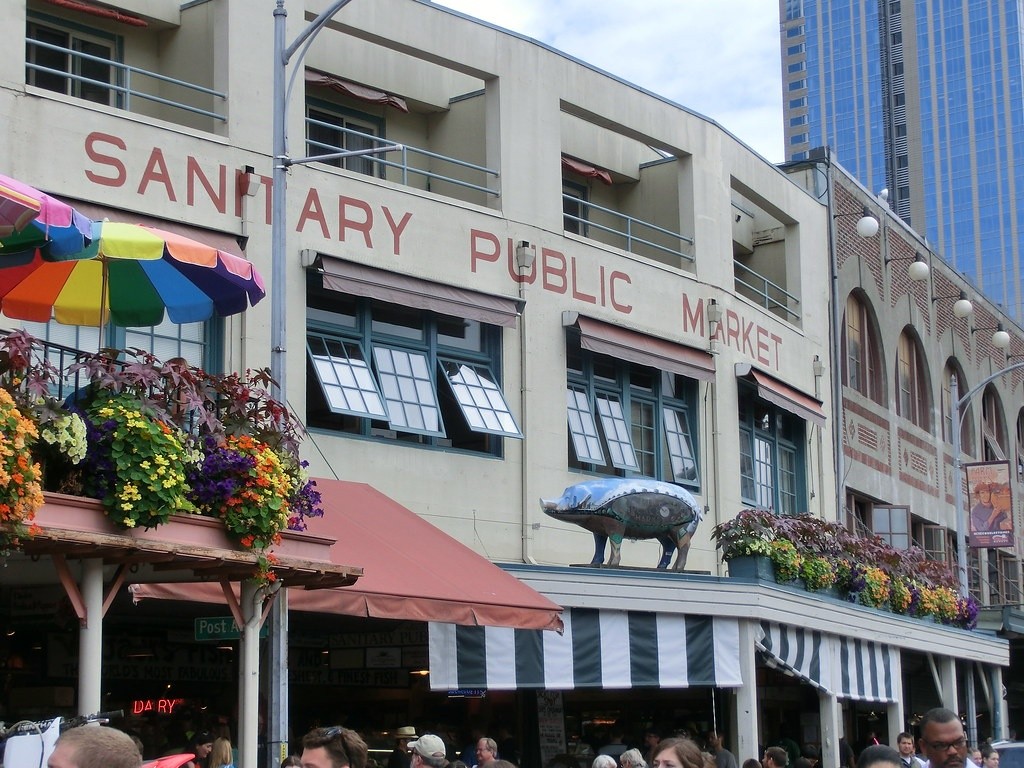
[46,709,1002,768]
[971,483,1007,532]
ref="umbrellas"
[0,173,92,268]
[0,218,266,355]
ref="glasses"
[476,747,488,752]
[922,738,968,751]
[199,729,209,736]
[322,728,353,768]
[411,739,417,741]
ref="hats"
[394,727,419,738]
[406,735,446,760]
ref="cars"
[979,741,1024,768]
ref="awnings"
[753,618,898,705]
[425,608,744,692]
[131,473,567,635]
[735,362,827,431]
[301,248,526,330]
[562,310,720,385]
